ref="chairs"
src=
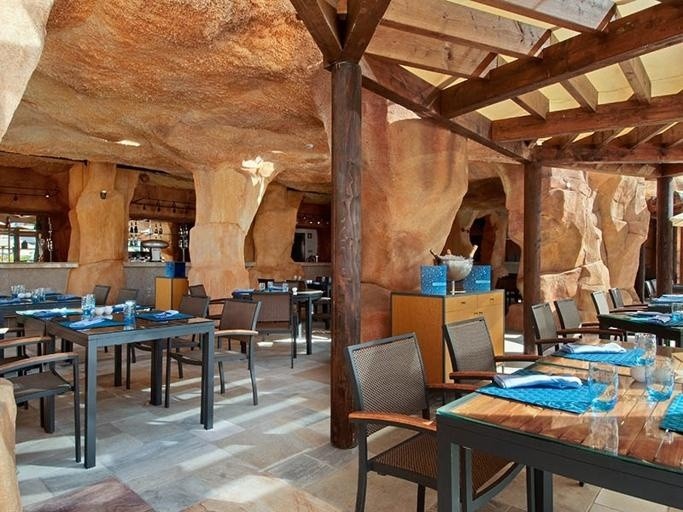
[0,283,263,469]
[257,276,332,369]
[441,279,657,487]
[343,332,535,512]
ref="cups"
[587,333,674,413]
[291,287,297,293]
[258,281,287,293]
[9,283,45,304]
[671,302,683,321]
[587,402,673,457]
[80,293,134,324]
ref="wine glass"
[432,257,473,296]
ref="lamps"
[99,190,107,200]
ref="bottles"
[429,249,440,260]
[464,244,479,260]
[127,219,189,262]
